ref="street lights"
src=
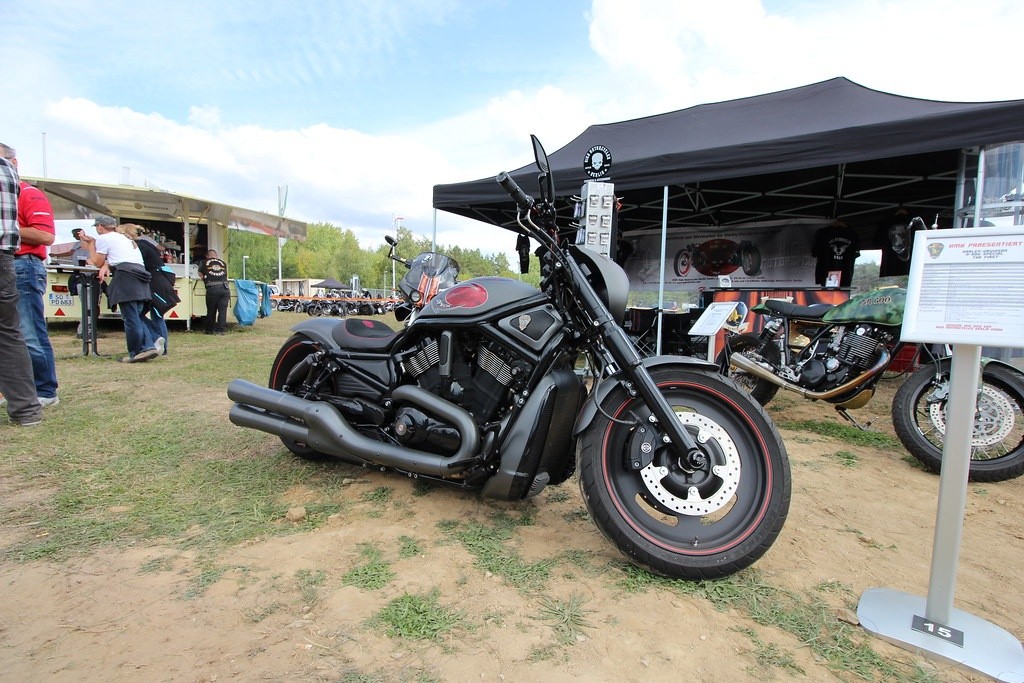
[242,256,250,281]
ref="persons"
[0,142,179,426]
[197,249,230,335]
[313,289,383,300]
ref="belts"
[15,253,39,259]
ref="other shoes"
[117,348,158,363]
[7,417,42,425]
[37,396,60,407]
[219,331,225,335]
[0,393,7,404]
[154,337,165,361]
[204,330,213,335]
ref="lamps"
[888,218,910,261]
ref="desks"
[43,264,114,360]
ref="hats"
[92,214,116,226]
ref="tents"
[432,76,1024,355]
[310,277,350,299]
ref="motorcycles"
[227,135,792,578]
[384,235,461,332]
[714,214,1024,482]
[277,291,404,319]
